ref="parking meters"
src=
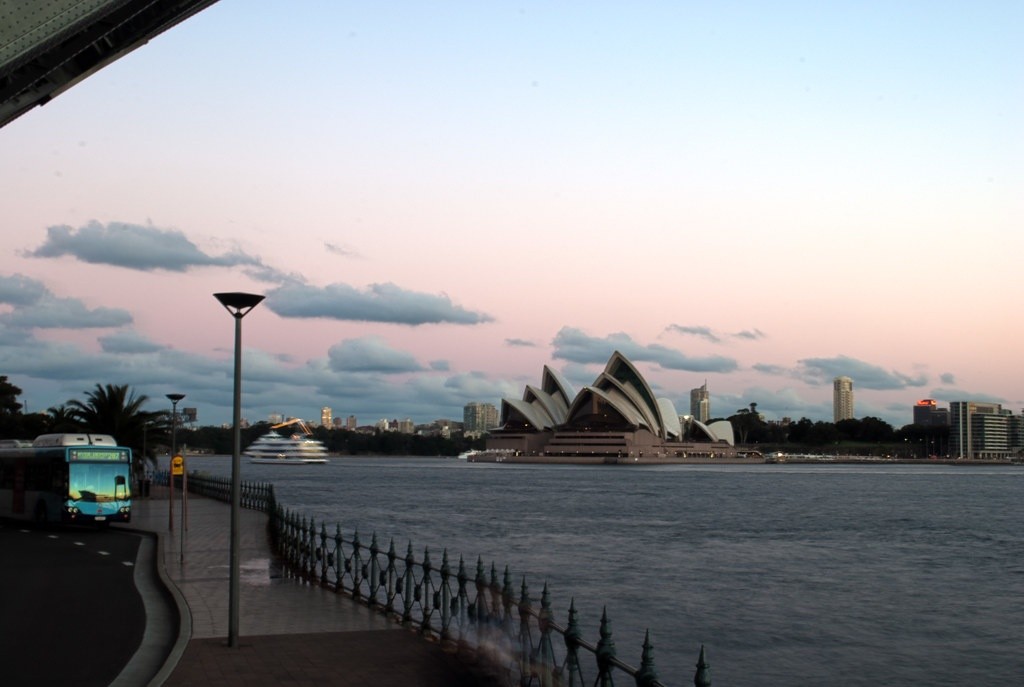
[171,454,188,531]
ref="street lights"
[213,291,268,648]
[165,391,186,533]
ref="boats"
[243,427,330,465]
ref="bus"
[0,434,133,532]
